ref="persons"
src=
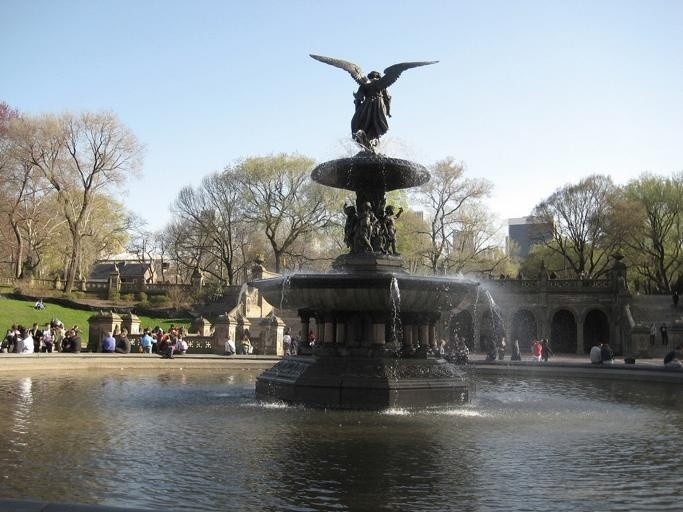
[223,334,235,355]
[541,339,554,363]
[497,335,507,360]
[0,316,190,361]
[238,333,254,356]
[600,342,614,365]
[282,331,290,357]
[485,340,497,360]
[510,339,521,361]
[649,321,657,346]
[33,298,44,309]
[590,342,604,365]
[487,268,651,295]
[307,328,316,347]
[659,322,670,346]
[36,300,44,310]
[341,202,356,256]
[379,205,404,255]
[533,338,542,361]
[351,200,379,257]
[349,70,392,155]
[672,290,679,308]
